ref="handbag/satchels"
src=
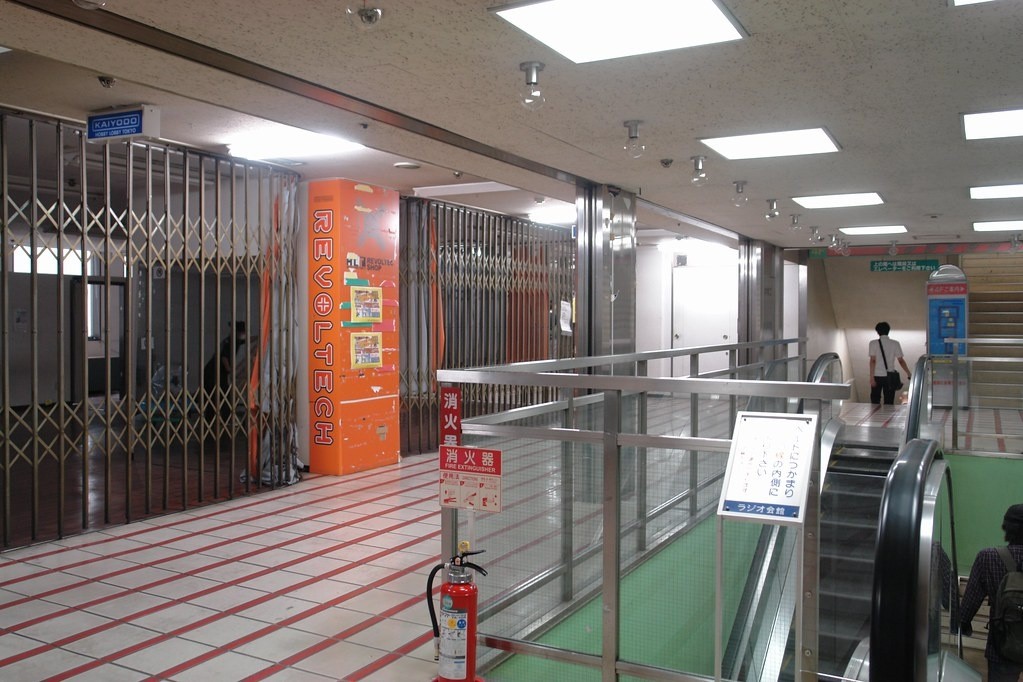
[886,370,904,390]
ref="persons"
[928,540,958,657]
[869,322,912,405]
[899,391,908,404]
[960,505,1023,682]
[204,322,258,436]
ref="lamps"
[8,175,104,199]
[63,145,224,188]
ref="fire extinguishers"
[425,539,490,682]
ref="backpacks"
[987,547,1023,665]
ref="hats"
[1003,504,1023,528]
